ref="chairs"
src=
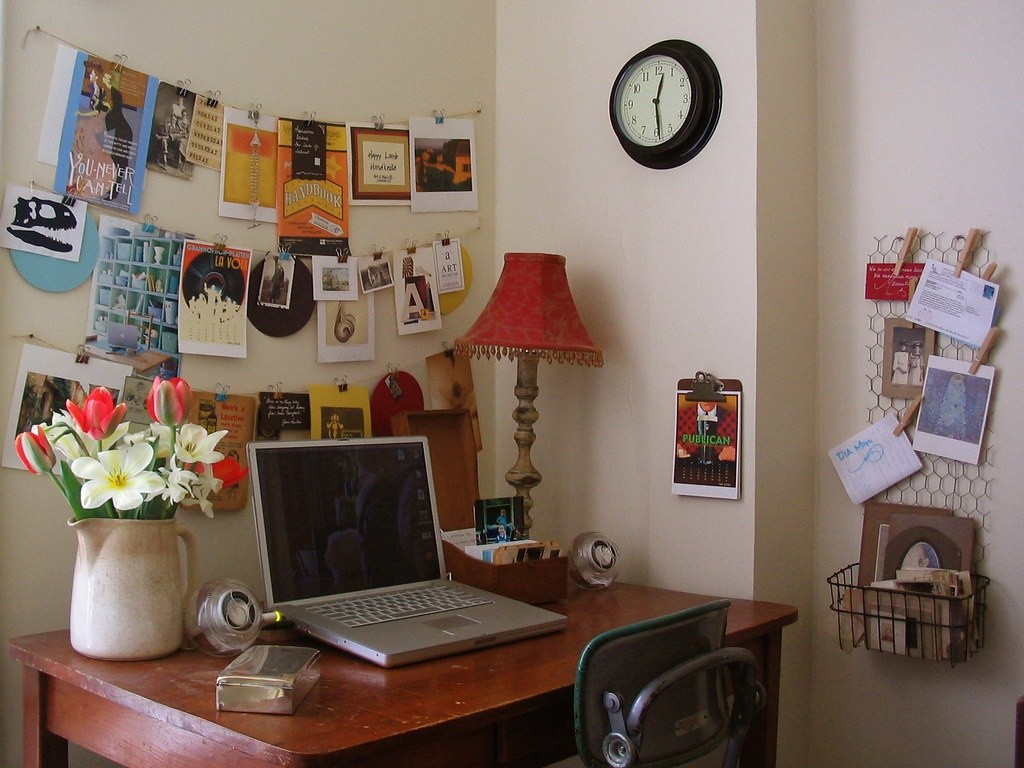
[572,598,758,767]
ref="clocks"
[610,39,722,170]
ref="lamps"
[453,253,609,539]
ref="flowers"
[14,374,229,519]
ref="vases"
[68,513,200,659]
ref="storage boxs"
[215,644,319,715]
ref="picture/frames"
[882,316,936,401]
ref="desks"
[11,576,799,768]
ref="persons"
[154,95,191,172]
[881,628,893,642]
[891,340,924,384]
[496,510,523,543]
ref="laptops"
[245,434,568,670]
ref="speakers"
[569,530,622,590]
[201,579,261,656]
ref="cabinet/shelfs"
[94,236,182,379]
